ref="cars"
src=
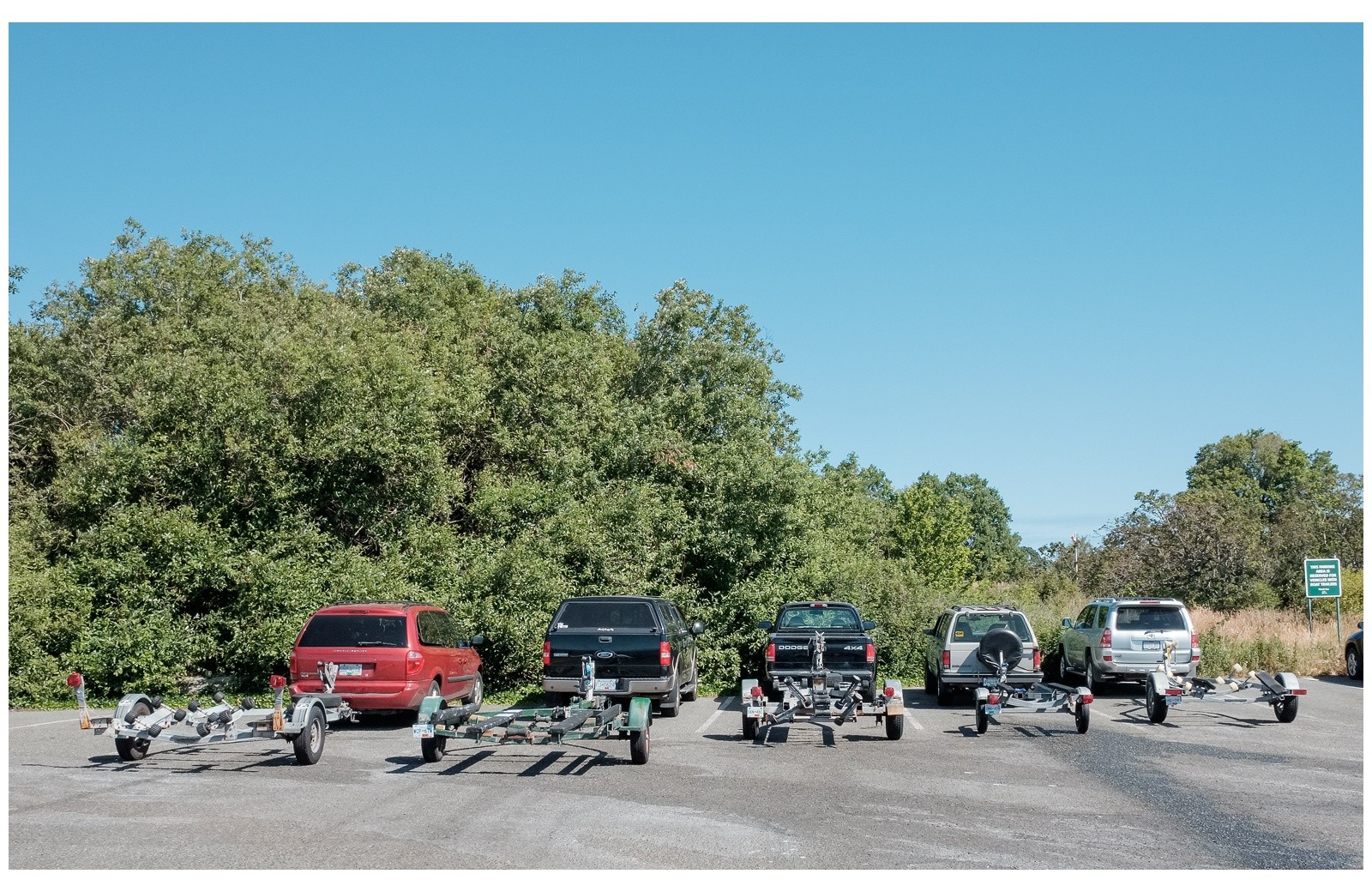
[1344,621,1363,681]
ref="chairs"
[1139,612,1155,622]
[786,616,805,625]
[830,619,845,626]
[954,622,972,637]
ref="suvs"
[921,604,1045,707]
[1056,596,1201,697]
[542,595,704,717]
[288,599,485,712]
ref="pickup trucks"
[759,601,878,703]
